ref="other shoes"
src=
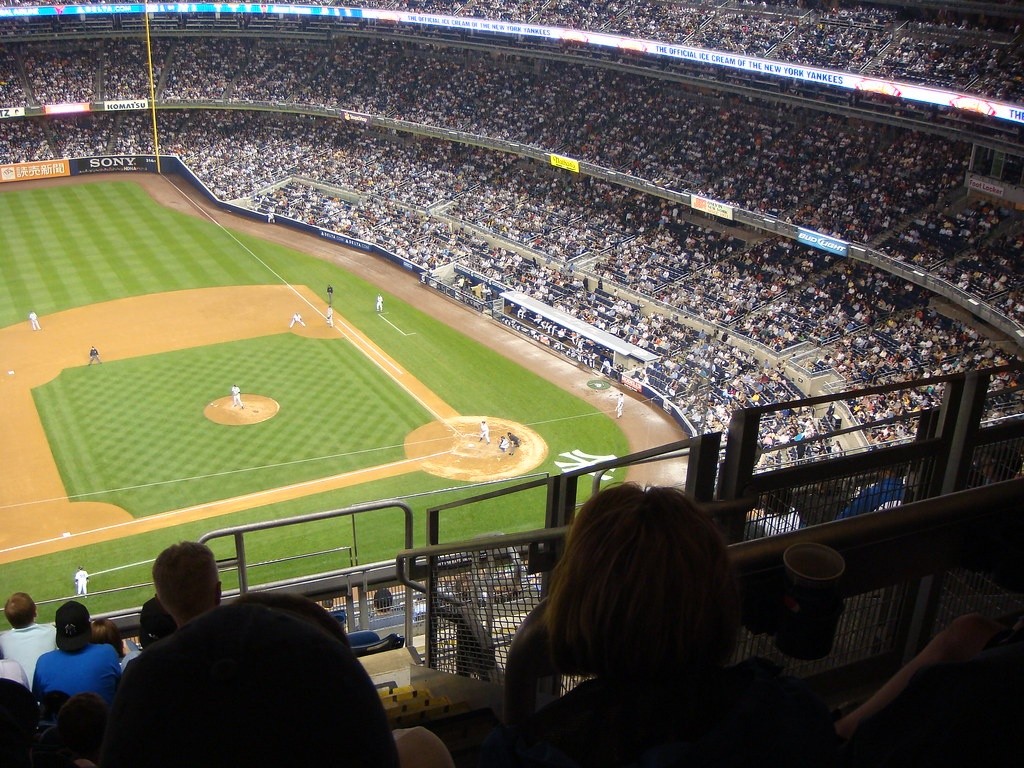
[509,453,513,455]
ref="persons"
[0,0,1024,476]
[453,437,1024,768]
[0,543,454,768]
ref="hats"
[55,601,92,651]
[620,393,623,395]
[508,432,511,435]
[482,421,486,423]
[501,436,505,439]
[79,566,83,570]
[138,594,177,649]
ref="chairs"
[346,628,405,657]
